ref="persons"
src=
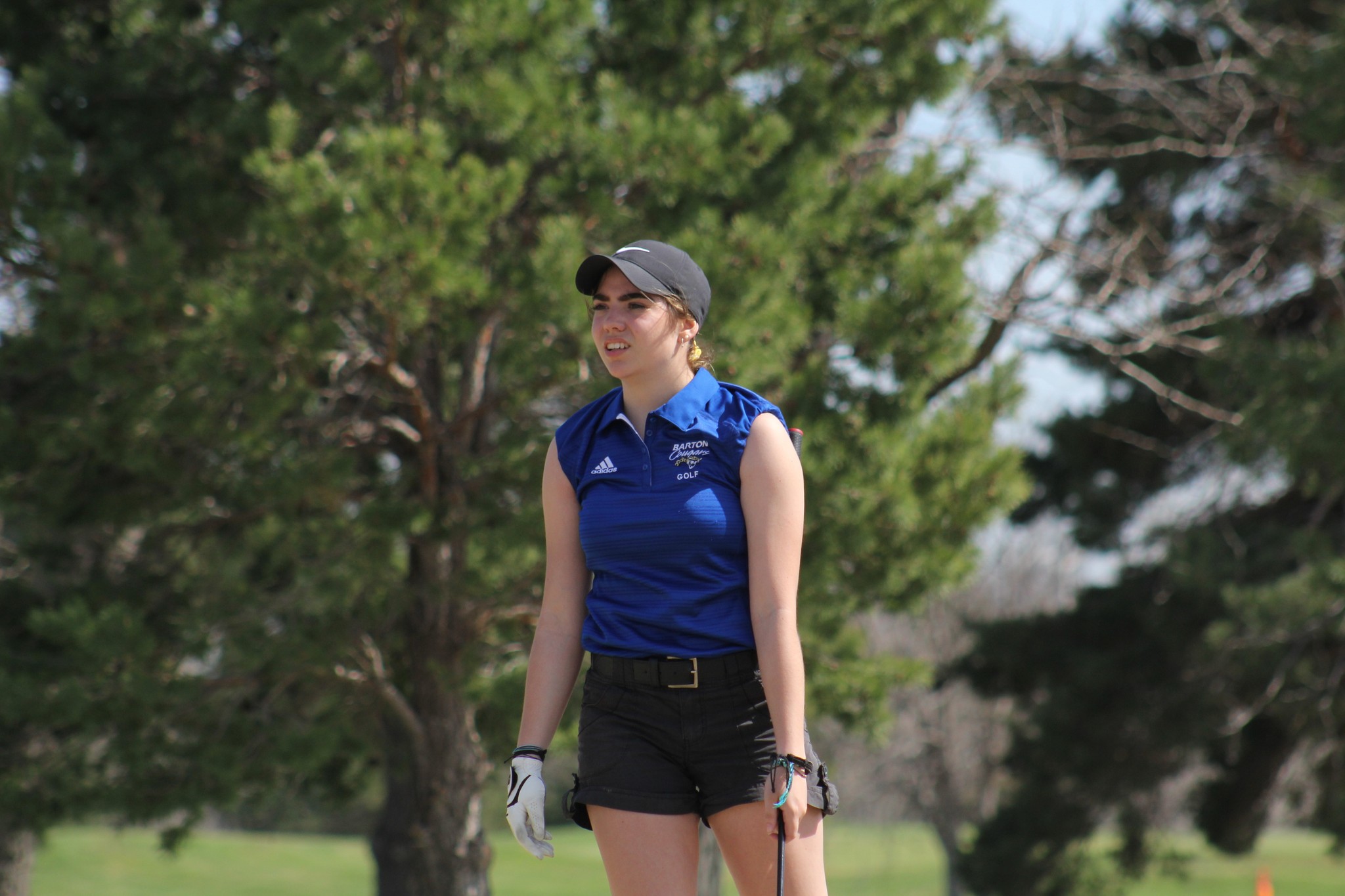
[503,240,838,895]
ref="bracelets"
[504,744,547,764]
[770,752,813,808]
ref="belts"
[594,651,753,688]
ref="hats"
[576,239,712,330]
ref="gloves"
[506,758,553,860]
